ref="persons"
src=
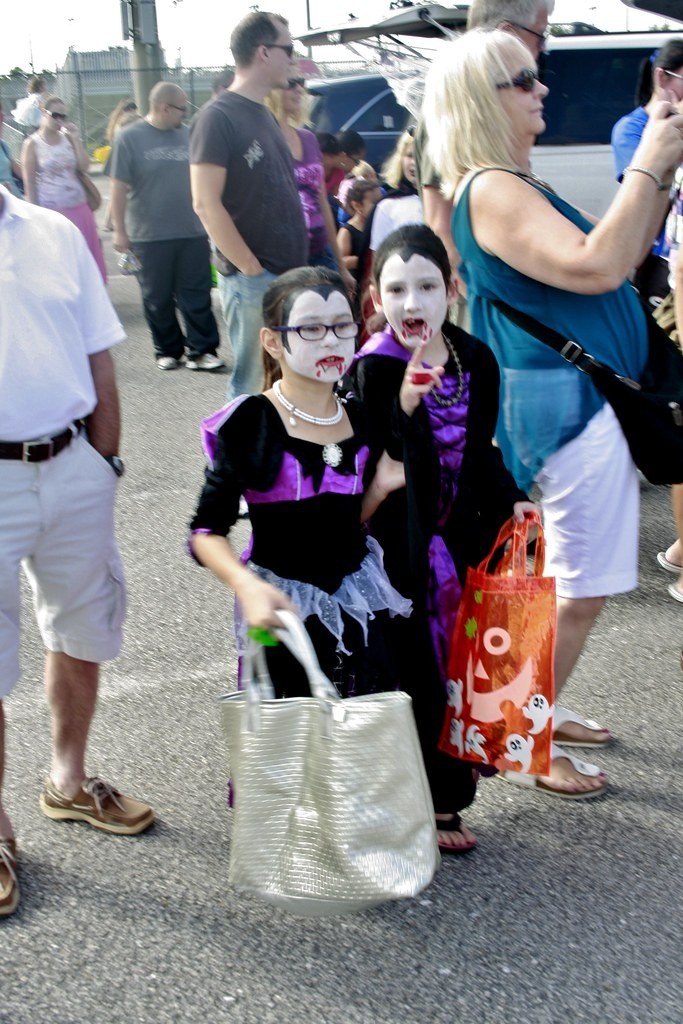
[186,265,406,699]
[612,39,683,183]
[187,12,311,402]
[424,29,683,799]
[0,183,155,913]
[348,224,538,850]
[0,138,23,198]
[413,0,555,334]
[657,247,683,605]
[21,97,107,283]
[317,128,424,300]
[21,77,48,138]
[262,63,356,295]
[110,82,226,370]
[103,101,142,231]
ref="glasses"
[503,18,550,51]
[166,102,188,113]
[496,69,542,93]
[273,321,359,341]
[253,43,294,60]
[43,108,67,123]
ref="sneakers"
[40,775,152,835]
[187,354,223,371]
[155,356,179,370]
[0,833,22,916]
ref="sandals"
[491,744,609,800]
[547,706,610,746]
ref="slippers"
[432,808,474,854]
[657,551,683,604]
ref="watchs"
[105,455,124,476]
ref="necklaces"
[273,379,342,428]
[430,332,463,407]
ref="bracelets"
[623,166,662,187]
[658,185,672,190]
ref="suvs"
[294,0,683,233]
[302,72,414,176]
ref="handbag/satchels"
[221,608,445,915]
[439,511,558,778]
[66,133,102,211]
[590,359,683,487]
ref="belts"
[0,416,87,462]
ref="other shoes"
[102,223,113,232]
